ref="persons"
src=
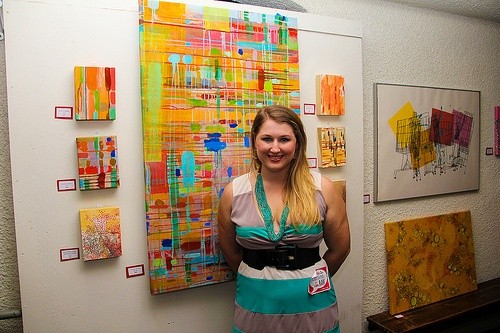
[218,105,350,333]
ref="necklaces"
[255,165,290,242]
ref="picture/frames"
[373,83,481,203]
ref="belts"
[242,245,321,271]
